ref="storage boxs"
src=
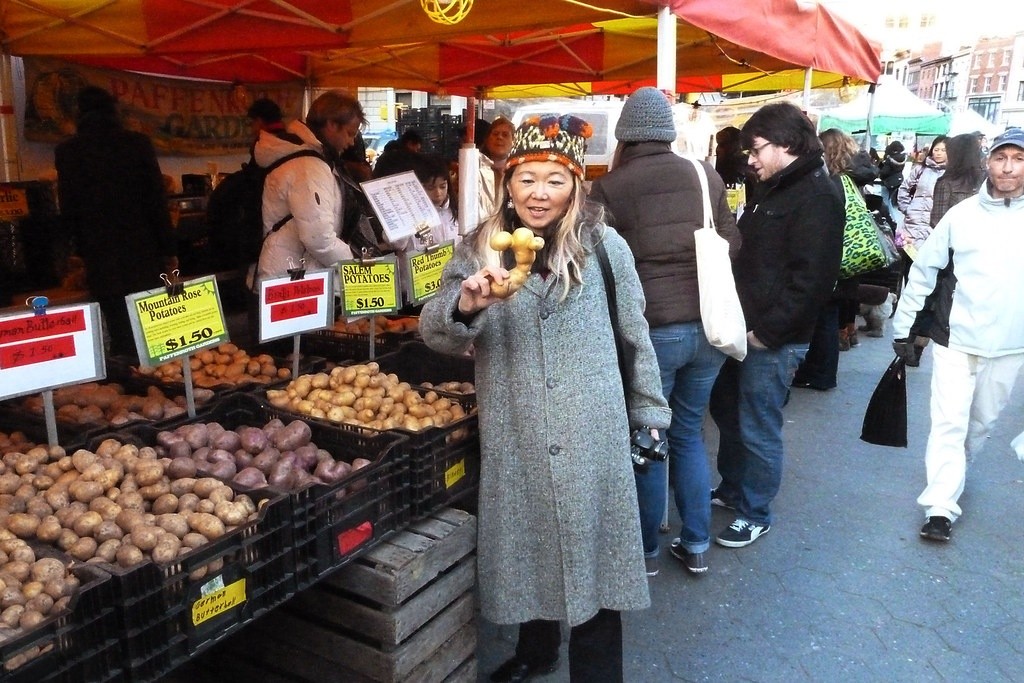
[0,287,481,683]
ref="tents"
[0,1,884,112]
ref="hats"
[990,128,1024,151]
[616,87,676,142]
[506,113,593,182]
[971,131,985,137]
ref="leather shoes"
[491,652,561,683]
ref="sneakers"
[716,517,770,547]
[920,515,951,542]
[642,555,658,576]
[670,536,708,573]
[710,486,736,509]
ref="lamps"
[838,76,857,102]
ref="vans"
[510,100,716,194]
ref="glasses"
[746,142,770,157]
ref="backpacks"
[206,150,334,271]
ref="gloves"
[893,342,916,368]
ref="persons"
[698,102,985,548]
[589,85,726,577]
[481,118,515,208]
[244,89,458,288]
[894,126,1024,545]
[418,116,672,683]
[55,86,179,323]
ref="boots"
[858,314,873,331]
[905,345,923,367]
[837,327,850,351]
[843,323,858,346]
[867,319,883,337]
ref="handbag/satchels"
[839,173,885,279]
[883,171,904,189]
[690,161,747,362]
[860,353,907,449]
[867,208,902,272]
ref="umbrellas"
[817,78,950,149]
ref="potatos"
[489,228,545,299]
[266,363,476,445]
[322,313,421,344]
[151,417,374,500]
[0,429,270,672]
[20,342,292,426]
